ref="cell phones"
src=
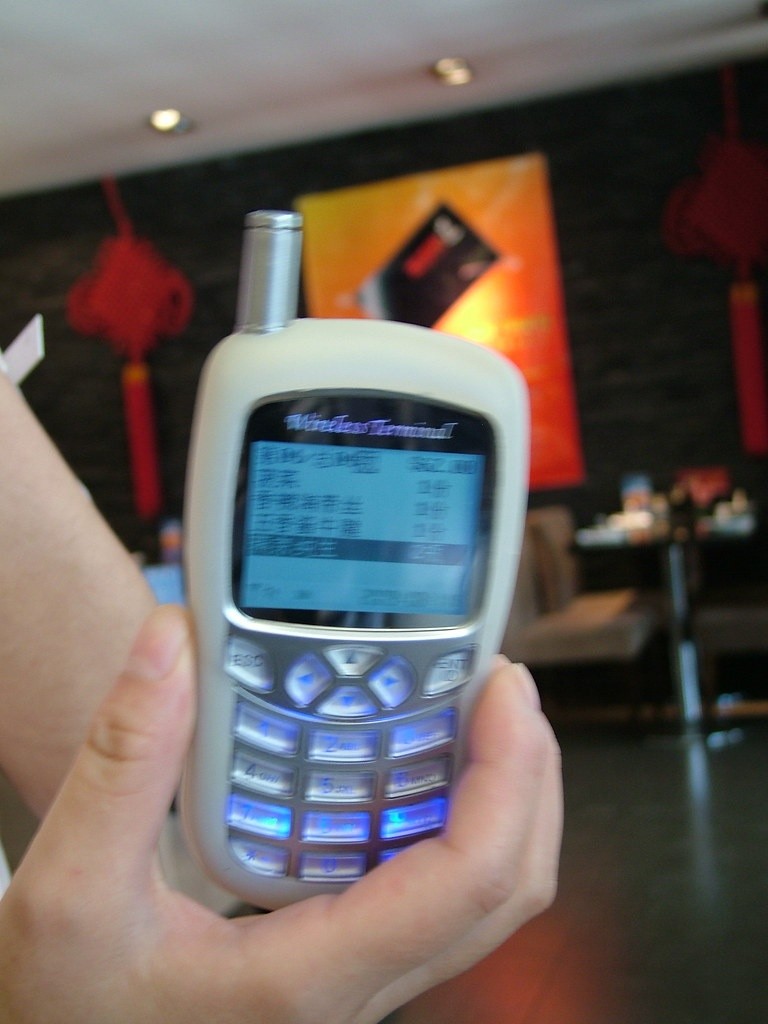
[175,210,531,909]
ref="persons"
[0,367,564,1024]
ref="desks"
[565,489,768,737]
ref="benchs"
[505,503,666,743]
